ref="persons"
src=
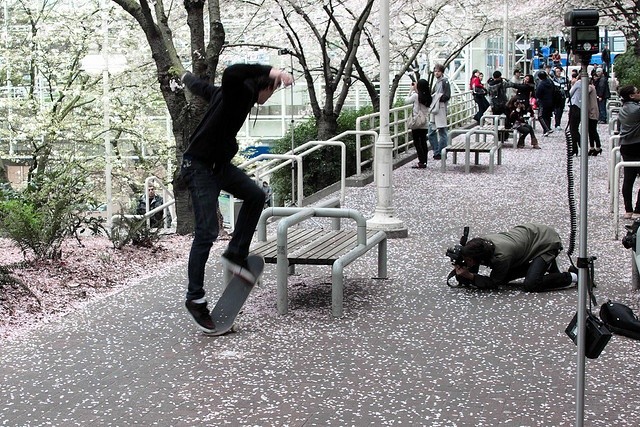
[510,70,523,93]
[136,185,172,228]
[533,48,540,68]
[261,181,272,208]
[455,222,598,292]
[565,35,572,65]
[566,70,582,157]
[513,75,535,117]
[544,64,554,79]
[591,63,598,76]
[591,71,599,85]
[428,64,451,159]
[596,68,611,123]
[552,49,561,66]
[588,80,602,156]
[601,44,612,78]
[536,71,554,137]
[552,67,570,131]
[617,85,639,221]
[478,73,483,82]
[404,79,432,169]
[540,43,550,68]
[470,69,490,122]
[528,91,539,111]
[180,64,294,334]
[505,94,541,150]
[486,71,513,126]
[599,64,603,68]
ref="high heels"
[570,149,578,156]
[588,148,595,156]
[592,149,602,156]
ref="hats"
[596,67,603,73]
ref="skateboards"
[203,254,265,336]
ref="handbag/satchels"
[473,85,488,96]
[407,108,426,129]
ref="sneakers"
[567,264,579,288]
[184,300,217,334]
[533,145,541,149]
[557,125,562,131]
[517,143,525,148]
[221,249,256,285]
[433,153,447,159]
[623,211,634,219]
[631,212,640,220]
[600,119,607,124]
[543,129,554,136]
[597,119,601,123]
[412,161,427,168]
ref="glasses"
[633,89,640,94]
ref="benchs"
[479,114,524,149]
[440,126,503,174]
[247,197,388,318]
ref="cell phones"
[412,82,415,86]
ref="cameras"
[447,227,472,268]
[564,7,600,57]
[623,232,636,250]
[563,308,611,359]
